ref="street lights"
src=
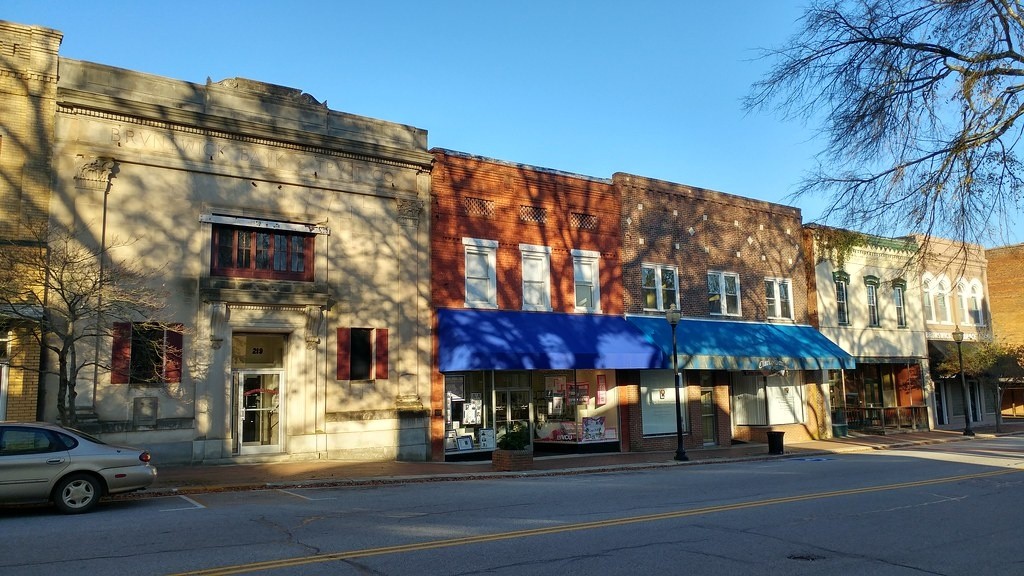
[952,325,976,436]
[664,304,691,462]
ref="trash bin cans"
[766,432,785,456]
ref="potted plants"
[492,425,533,471]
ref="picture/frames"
[446,429,474,451]
[445,375,465,401]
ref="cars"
[0,420,158,515]
